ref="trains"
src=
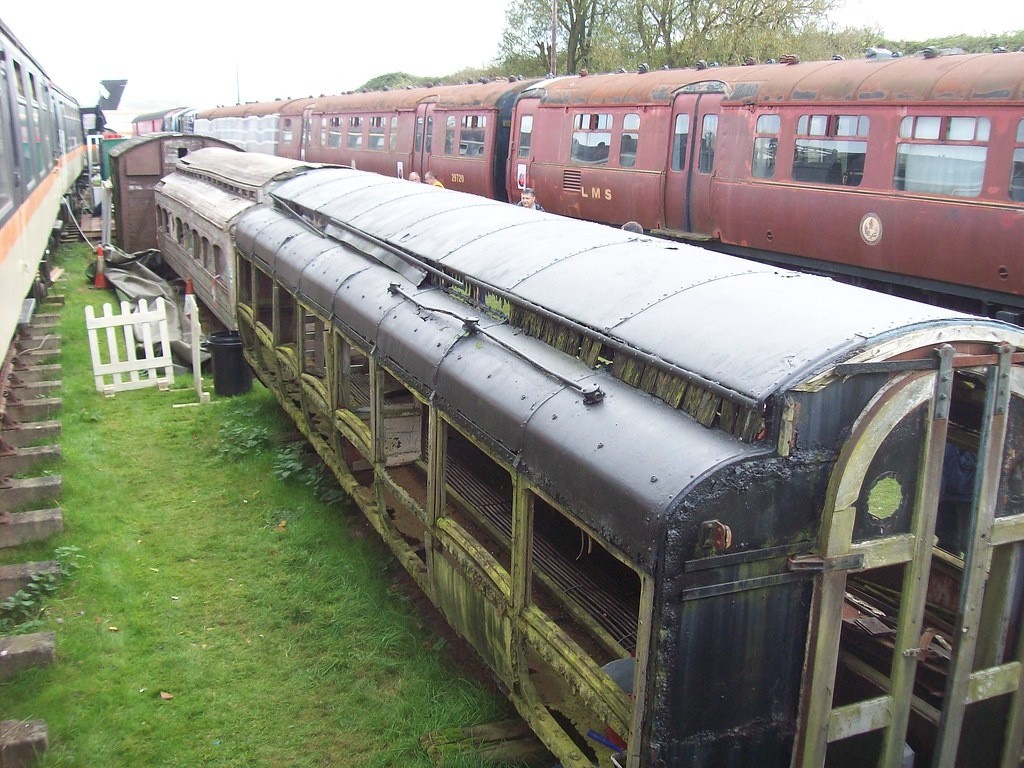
[0,19,1024,768]
